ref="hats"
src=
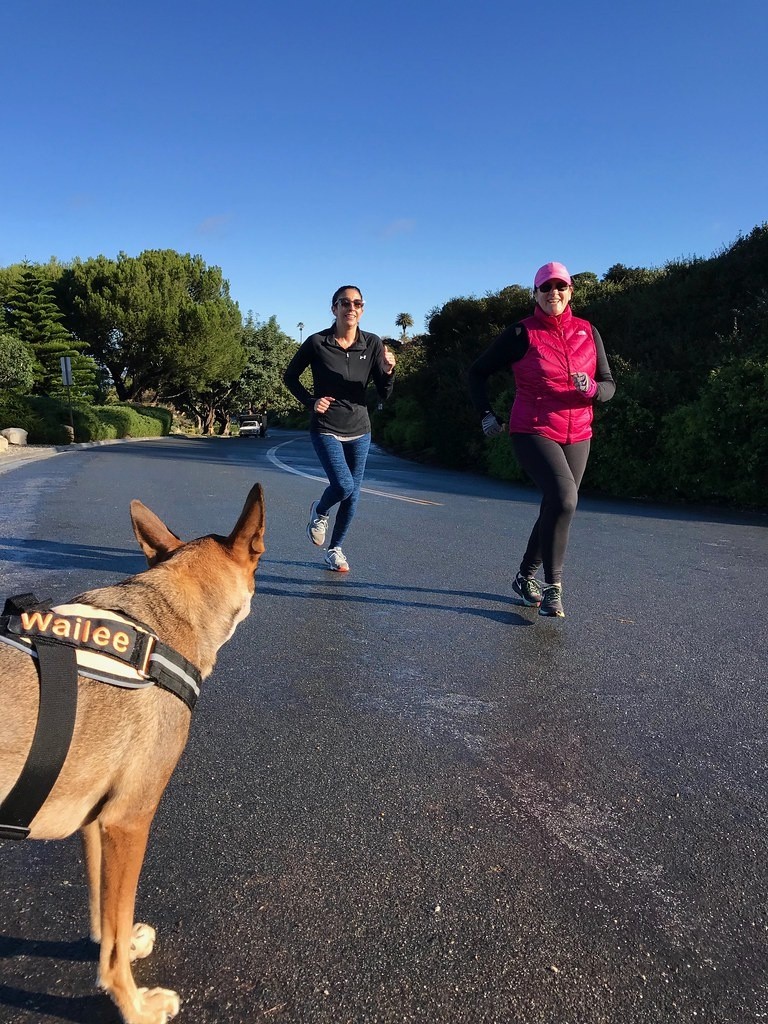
[534,262,571,289]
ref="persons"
[488,261,617,617]
[283,286,396,571]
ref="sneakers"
[512,571,543,607]
[325,547,349,571]
[539,584,565,617]
[306,501,330,546]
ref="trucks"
[236,413,268,439]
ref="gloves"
[574,372,588,391]
[482,413,502,437]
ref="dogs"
[0,482,267,1023]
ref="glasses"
[334,298,364,308]
[537,281,568,293]
